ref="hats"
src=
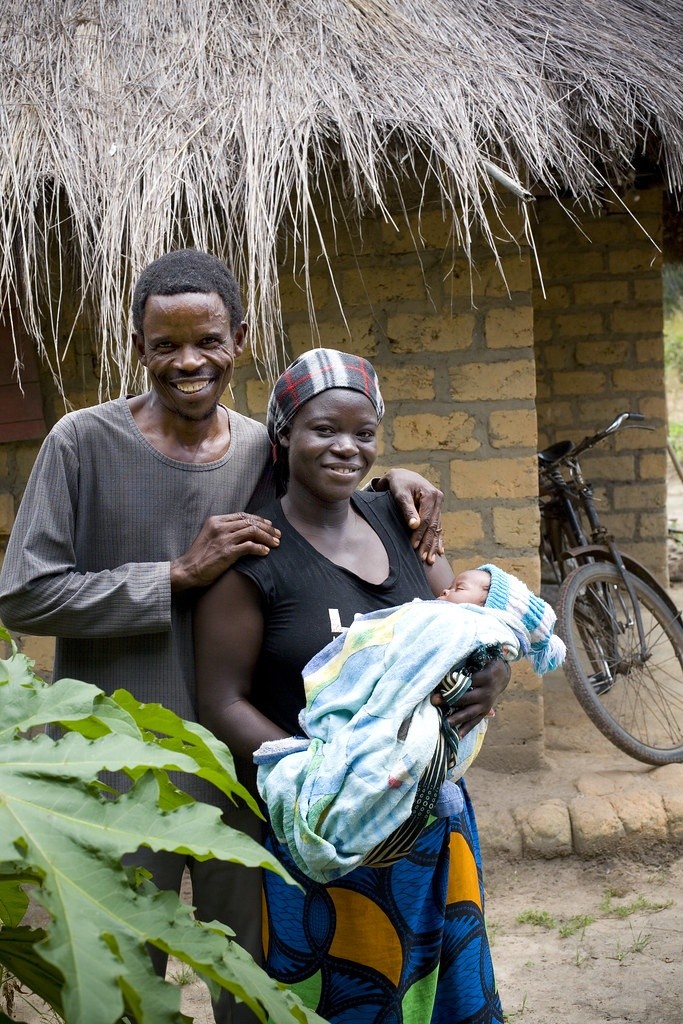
[475,563,566,678]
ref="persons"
[193,344,511,1024]
[0,249,445,1024]
[253,564,569,885]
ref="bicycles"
[537,412,683,766]
